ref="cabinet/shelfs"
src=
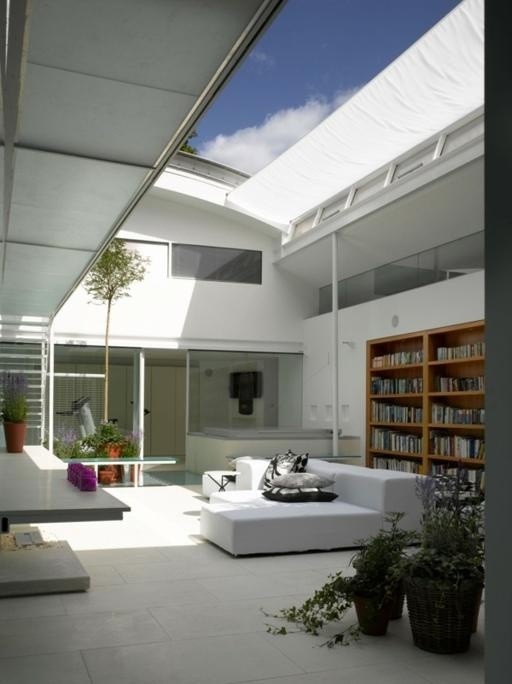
[362,320,484,474]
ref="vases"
[3,420,27,453]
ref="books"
[368,340,486,506]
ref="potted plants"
[86,422,130,458]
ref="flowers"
[0,369,32,422]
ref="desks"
[1,444,132,597]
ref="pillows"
[261,448,340,503]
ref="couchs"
[201,457,431,558]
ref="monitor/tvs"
[230,372,258,398]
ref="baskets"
[405,576,472,655]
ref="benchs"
[62,456,177,486]
